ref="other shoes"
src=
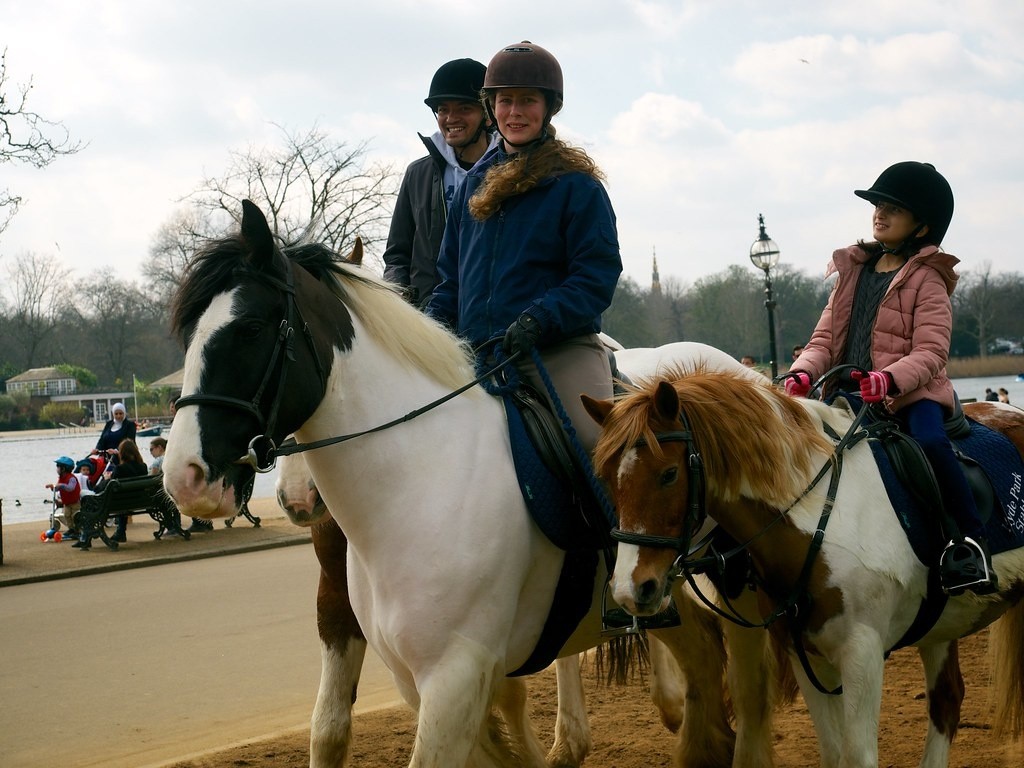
[185,522,214,532]
[62,528,73,537]
[71,541,92,548]
[602,604,681,630]
[67,533,80,539]
[109,533,126,542]
[942,538,999,595]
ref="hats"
[111,402,126,432]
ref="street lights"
[750,213,781,384]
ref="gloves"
[852,370,891,401]
[504,317,540,359]
[784,370,813,398]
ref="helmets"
[55,457,74,468]
[424,57,491,99]
[854,159,954,219]
[482,40,563,90]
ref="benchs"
[72,474,260,550]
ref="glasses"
[115,412,123,415]
[150,446,158,451]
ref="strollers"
[71,449,116,493]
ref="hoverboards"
[40,484,80,542]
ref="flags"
[134,378,144,388]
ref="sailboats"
[132,374,165,437]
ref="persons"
[792,345,804,362]
[784,160,999,594]
[90,405,137,524]
[420,41,684,627]
[1018,370,1024,378]
[169,395,214,532]
[76,459,92,474]
[148,438,181,536]
[45,457,80,541]
[741,353,767,376]
[984,387,1009,406]
[71,439,149,547]
[382,58,501,314]
[132,417,149,429]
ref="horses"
[159,199,800,766]
[579,358,1024,768]
